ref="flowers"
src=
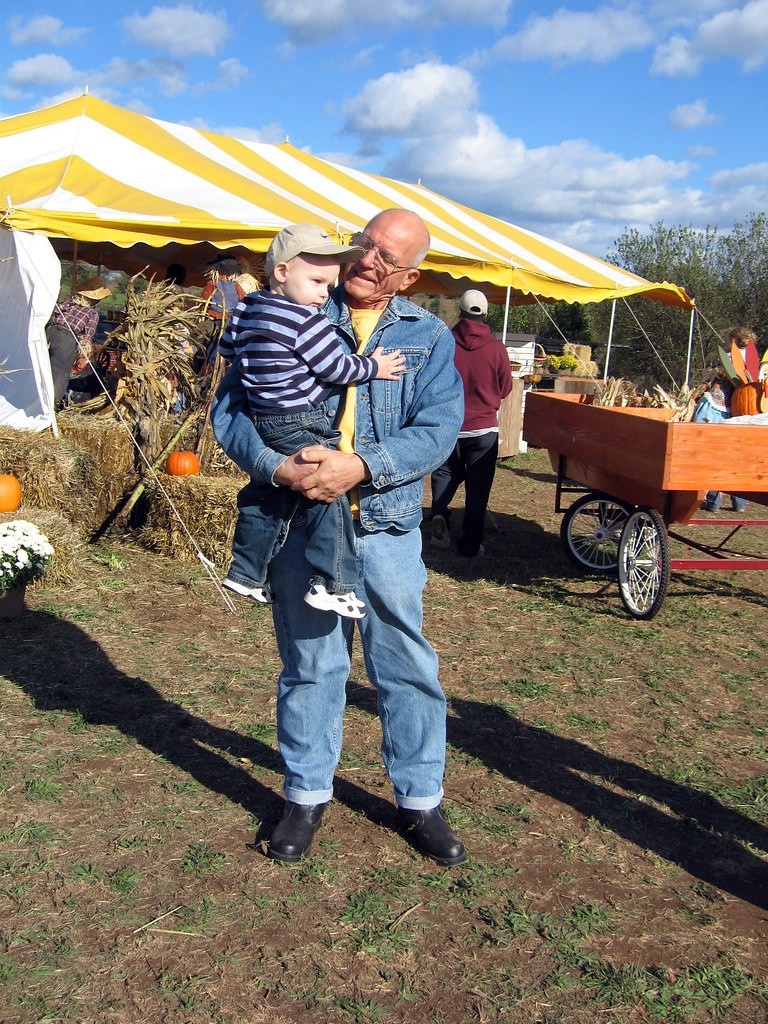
[541,354,578,373]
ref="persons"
[218,224,408,619]
[702,327,758,512]
[208,208,470,864]
[200,253,246,386]
[45,276,112,405]
[428,289,514,560]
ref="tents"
[0,84,699,389]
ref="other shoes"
[734,505,746,514]
[701,501,719,514]
[432,515,453,551]
[478,545,486,556]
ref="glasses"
[350,230,415,277]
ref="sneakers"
[304,584,366,619]
[221,575,275,604]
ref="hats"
[74,275,111,301]
[263,225,365,281]
[459,290,488,316]
[207,255,243,276]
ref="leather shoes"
[397,805,471,865]
[270,799,325,862]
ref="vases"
[519,376,530,384]
[534,358,548,365]
[510,361,522,371]
[528,372,542,383]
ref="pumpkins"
[167,451,199,475]
[0,466,21,513]
[731,370,763,417]
